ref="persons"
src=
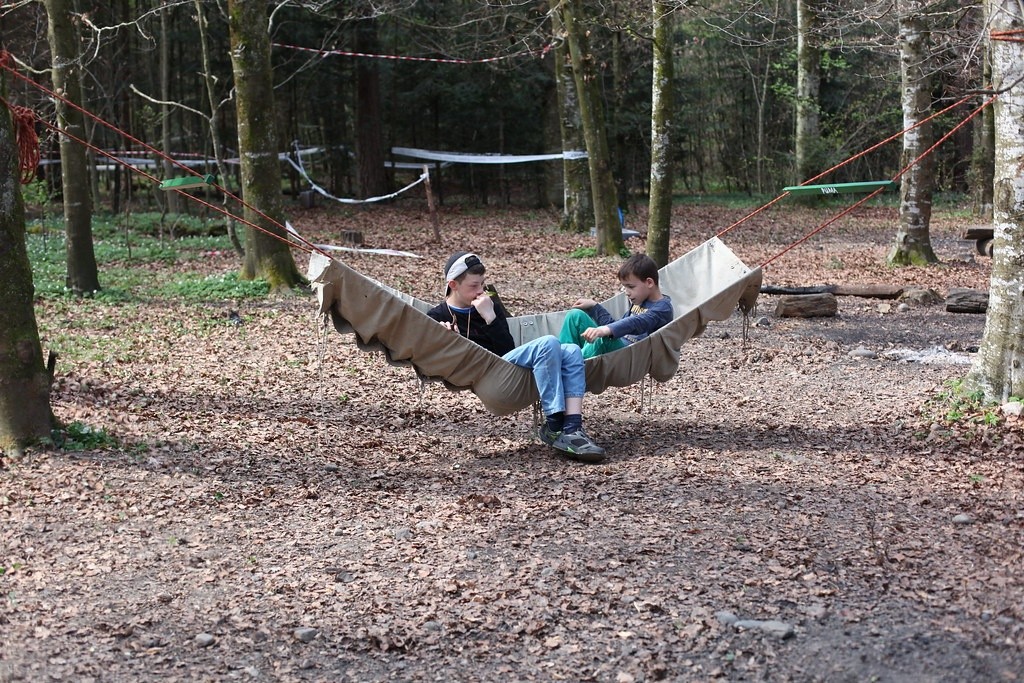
[556,253,673,359]
[424,250,606,462]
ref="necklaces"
[446,301,470,339]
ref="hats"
[444,252,482,298]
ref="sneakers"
[539,422,562,445]
[553,431,606,460]
